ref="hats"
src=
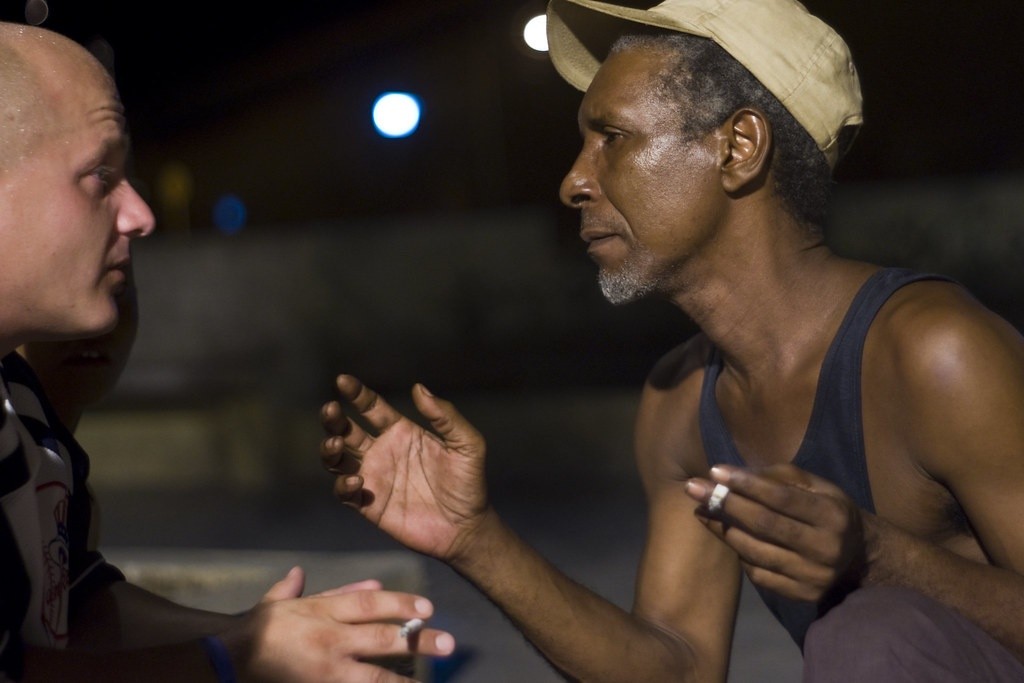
[546,0,864,169]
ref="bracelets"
[201,635,236,683]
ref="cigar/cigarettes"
[400,618,427,636]
[708,483,729,510]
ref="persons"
[0,22,455,683]
[321,0,1024,683]
